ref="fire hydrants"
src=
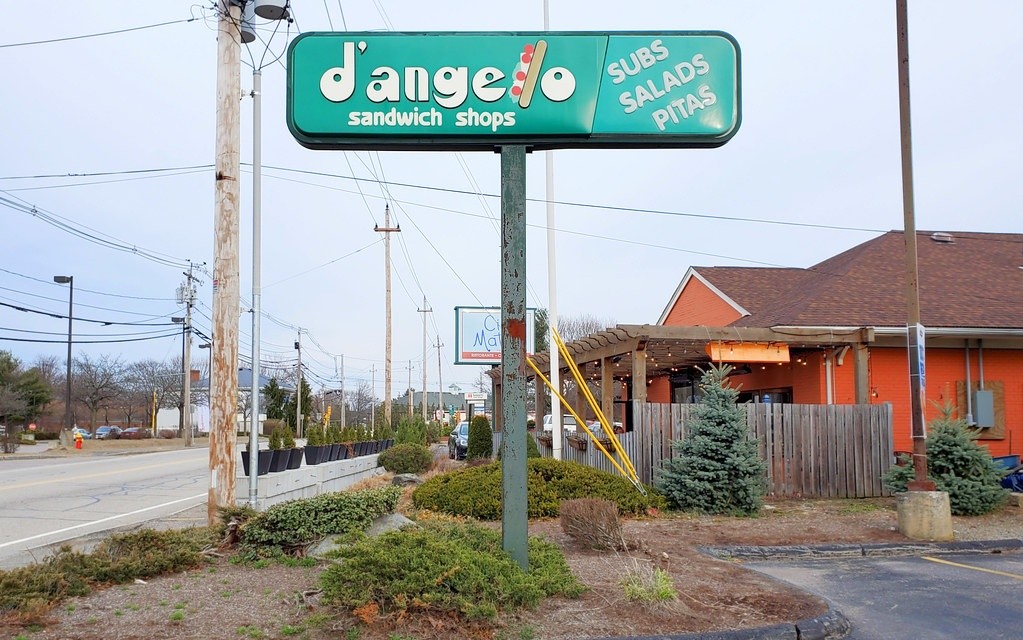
[74,434,83,449]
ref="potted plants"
[241,430,275,475]
[282,424,305,469]
[304,417,395,465]
[267,424,292,471]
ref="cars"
[95,426,120,440]
[121,427,149,440]
[71,429,92,440]
[447,421,473,461]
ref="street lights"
[171,316,188,437]
[54,275,75,447]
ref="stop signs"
[29,424,36,430]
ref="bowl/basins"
[993,454,1020,470]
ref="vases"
[593,438,617,452]
[537,435,553,446]
[566,435,587,450]
